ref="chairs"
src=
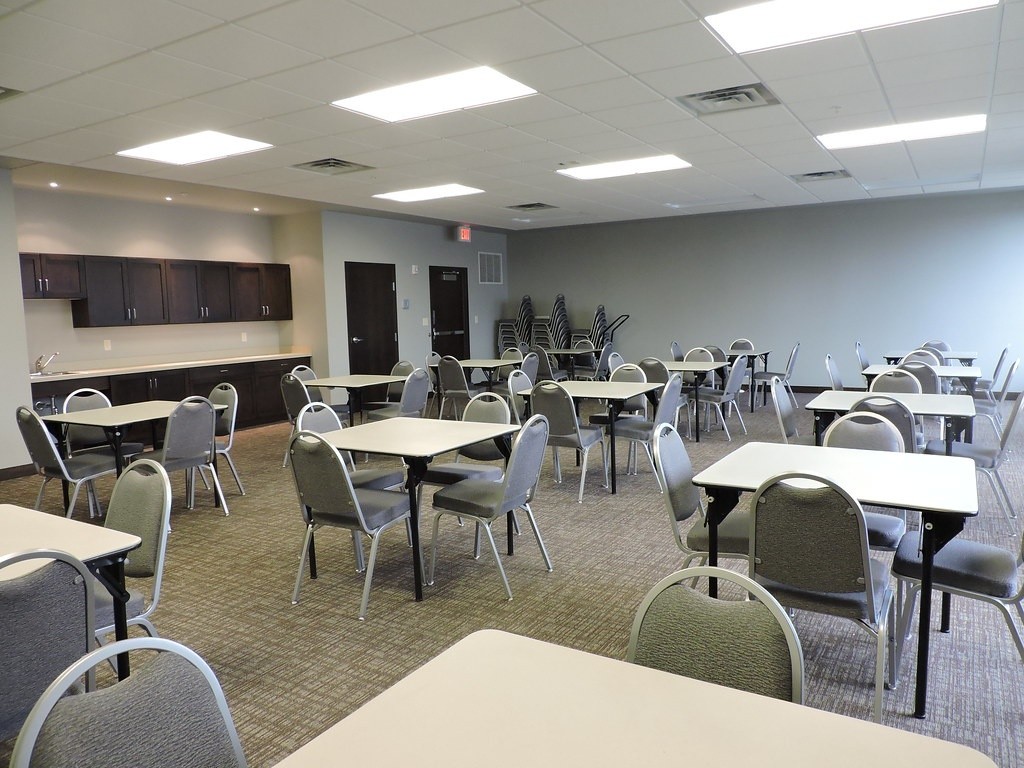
[0,292,1024,768]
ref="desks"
[288,413,523,608]
[883,350,979,366]
[692,440,981,720]
[517,380,666,493]
[425,358,522,421]
[37,398,230,517]
[861,364,983,394]
[0,504,146,682]
[302,373,407,468]
[273,626,1003,768]
[724,349,773,413]
[546,348,602,380]
[801,389,977,454]
[648,360,731,440]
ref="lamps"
[455,225,472,243]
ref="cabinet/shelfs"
[253,358,311,423]
[70,253,168,328]
[19,252,86,301]
[233,262,293,322]
[190,359,257,429]
[109,369,189,444]
[165,258,234,324]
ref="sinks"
[30,371,78,377]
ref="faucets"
[35,352,59,372]
